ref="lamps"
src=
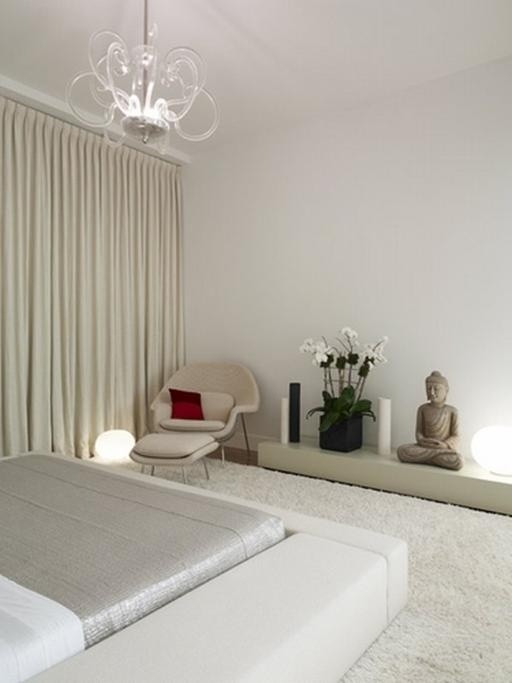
[89,429,136,462]
[472,425,512,479]
[66,1,221,150]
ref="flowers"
[293,328,387,428]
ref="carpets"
[118,444,512,682]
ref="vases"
[311,413,367,452]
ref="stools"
[131,435,220,485]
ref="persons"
[397,371,463,471]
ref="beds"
[1,444,411,683]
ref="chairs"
[144,359,259,470]
